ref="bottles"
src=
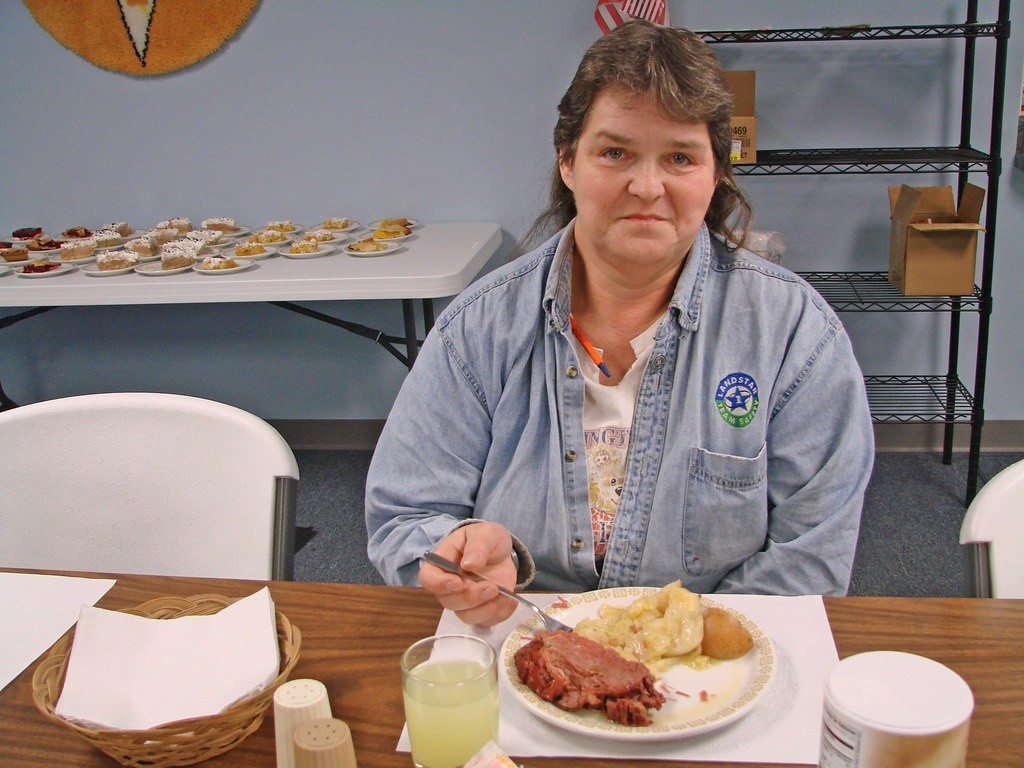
[274,678,333,768]
[818,649,975,768]
[294,718,358,768]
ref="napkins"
[52,582,281,743]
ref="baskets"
[31,591,302,768]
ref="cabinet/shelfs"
[630,0,1013,510]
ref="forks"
[424,551,574,633]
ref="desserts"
[0,217,413,274]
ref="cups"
[401,634,499,768]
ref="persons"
[365,15,875,627]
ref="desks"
[0,220,502,414]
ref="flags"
[594,0,672,35]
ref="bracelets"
[511,546,520,571]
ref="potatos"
[701,608,753,660]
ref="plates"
[279,243,336,259]
[296,233,348,243]
[221,247,276,260]
[359,231,413,241]
[253,224,304,233]
[309,221,360,233]
[499,587,776,742]
[367,218,419,228]
[240,235,293,247]
[343,242,402,256]
[0,223,256,278]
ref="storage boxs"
[887,181,986,296]
[723,70,758,165]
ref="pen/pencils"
[570,316,611,377]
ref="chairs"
[0,390,300,581]
[958,458,1024,599]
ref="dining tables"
[0,567,1024,768]
